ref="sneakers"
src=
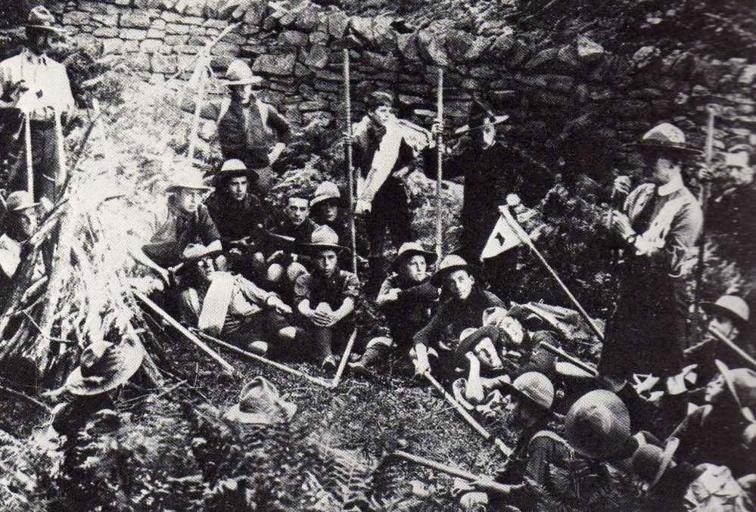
[321,355,336,370]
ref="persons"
[419,98,555,307]
[178,47,292,185]
[0,187,55,291]
[0,4,77,187]
[597,122,704,384]
[701,143,755,349]
[125,155,754,511]
[49,340,149,511]
[342,90,419,293]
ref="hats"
[631,437,680,492]
[169,159,259,276]
[61,339,143,397]
[453,305,554,411]
[566,390,632,461]
[5,189,41,213]
[454,100,509,136]
[716,358,755,425]
[698,296,753,334]
[619,122,703,155]
[220,377,297,427]
[24,6,67,33]
[221,61,263,87]
[301,225,476,286]
[308,180,340,206]
